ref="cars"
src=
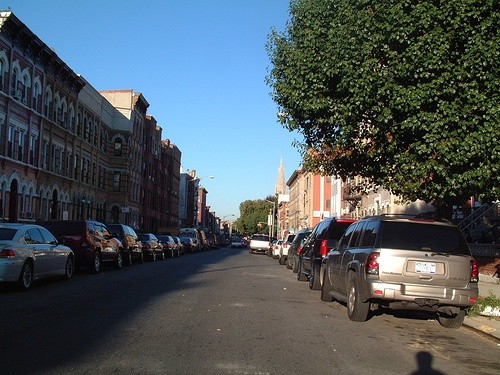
[142,233,165,262]
[0,222,76,289]
[231,237,248,249]
[268,239,283,260]
[172,228,232,256]
[156,236,180,259]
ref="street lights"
[184,176,216,228]
[223,214,234,224]
[257,195,275,238]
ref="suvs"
[249,234,272,255]
[279,234,296,265]
[320,213,480,329]
[103,223,144,267]
[286,227,312,273]
[297,216,358,290]
[53,219,125,274]
